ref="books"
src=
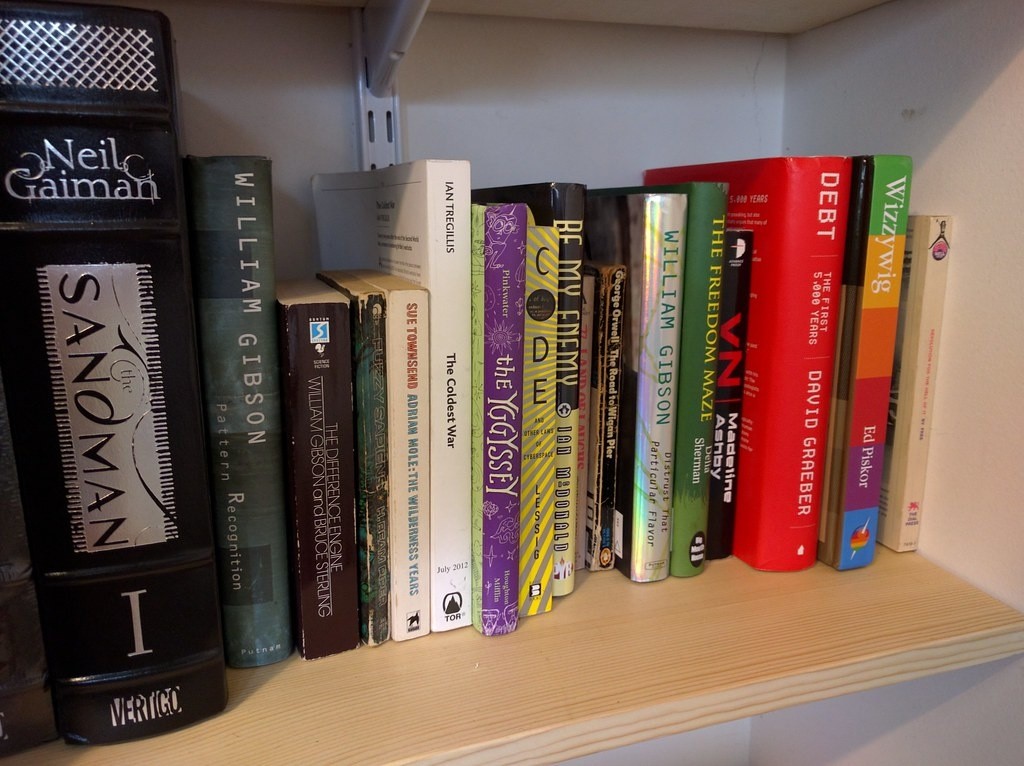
[0,0,953,760]
